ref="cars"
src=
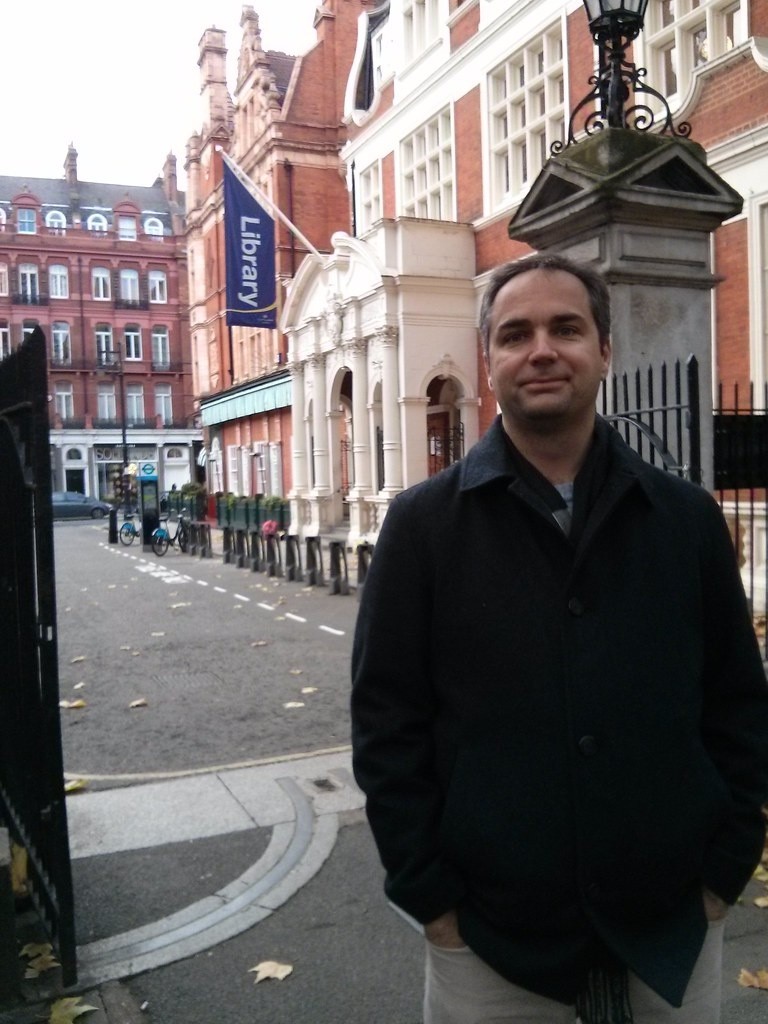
[51,492,114,520]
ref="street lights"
[96,342,133,521]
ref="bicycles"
[150,507,190,557]
[120,508,141,546]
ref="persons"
[352,253,768,1024]
